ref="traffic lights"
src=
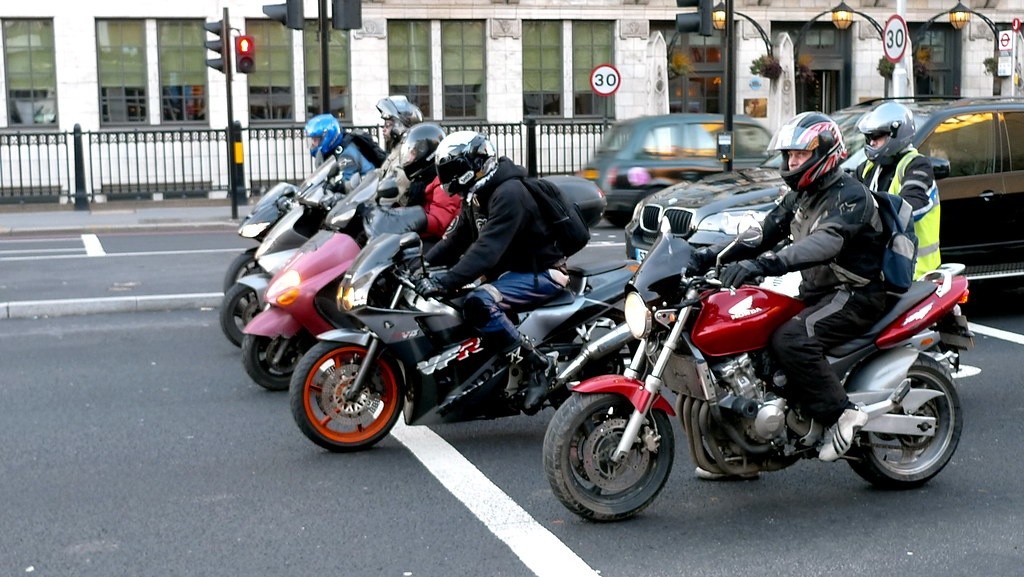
[203,20,225,72]
[235,36,256,73]
[262,0,304,30]
[676,0,713,36]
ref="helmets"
[426,132,498,197]
[780,112,847,192]
[864,103,914,161]
[377,94,423,143]
[305,113,344,158]
[399,123,446,181]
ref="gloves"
[719,250,787,288]
[414,276,448,301]
[686,245,717,276]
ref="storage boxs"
[540,176,607,228]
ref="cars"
[580,113,774,229]
[669,81,706,113]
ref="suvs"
[625,95,1024,291]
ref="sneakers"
[818,403,868,462]
[693,457,759,481]
[523,355,558,416]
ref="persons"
[695,111,917,480]
[851,102,942,283]
[415,131,593,415]
[305,116,386,184]
[396,124,470,258]
[342,95,429,208]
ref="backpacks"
[340,128,386,168]
[828,175,918,293]
[520,175,590,257]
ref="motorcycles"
[221,154,341,351]
[542,216,969,523]
[289,232,653,452]
[241,171,608,391]
[919,262,975,374]
[223,182,299,319]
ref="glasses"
[865,132,887,141]
[384,122,393,128]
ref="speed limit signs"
[590,65,621,97]
[883,15,907,63]
